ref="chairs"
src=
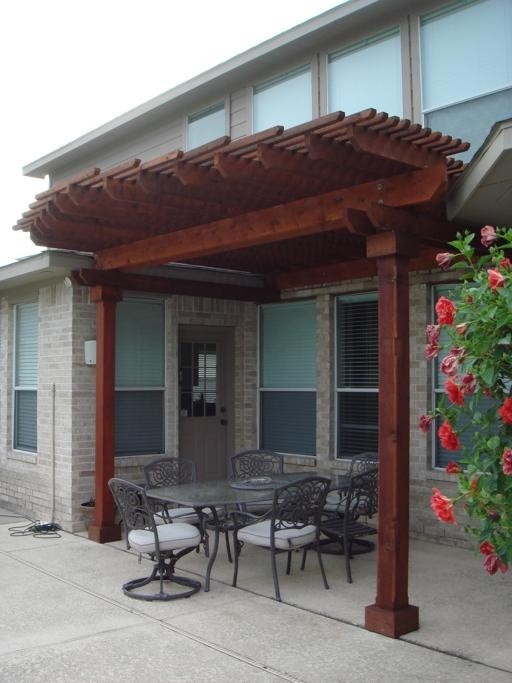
[232,475,331,600]
[229,451,285,555]
[320,450,378,584]
[144,459,233,563]
[108,478,202,600]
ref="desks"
[145,472,366,593]
